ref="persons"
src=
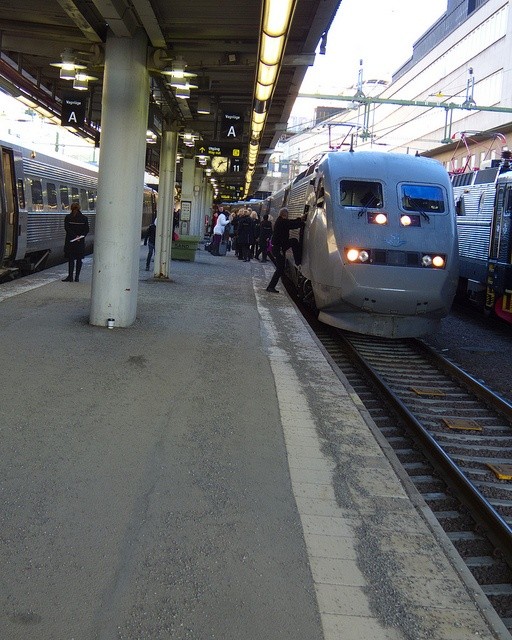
[250,210,259,225]
[174,209,179,230]
[61,202,89,282]
[255,214,272,262]
[247,208,252,213]
[238,210,253,260]
[144,217,157,271]
[230,210,237,250]
[230,208,244,256]
[266,207,307,293]
[210,210,229,255]
[211,210,218,228]
[218,206,229,215]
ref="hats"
[71,203,81,210]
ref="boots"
[75,262,82,281]
[61,262,74,282]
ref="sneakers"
[267,286,279,293]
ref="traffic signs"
[194,140,241,157]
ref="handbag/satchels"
[173,231,179,240]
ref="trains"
[449,151,512,324]
[0,139,158,280]
[220,151,460,339]
